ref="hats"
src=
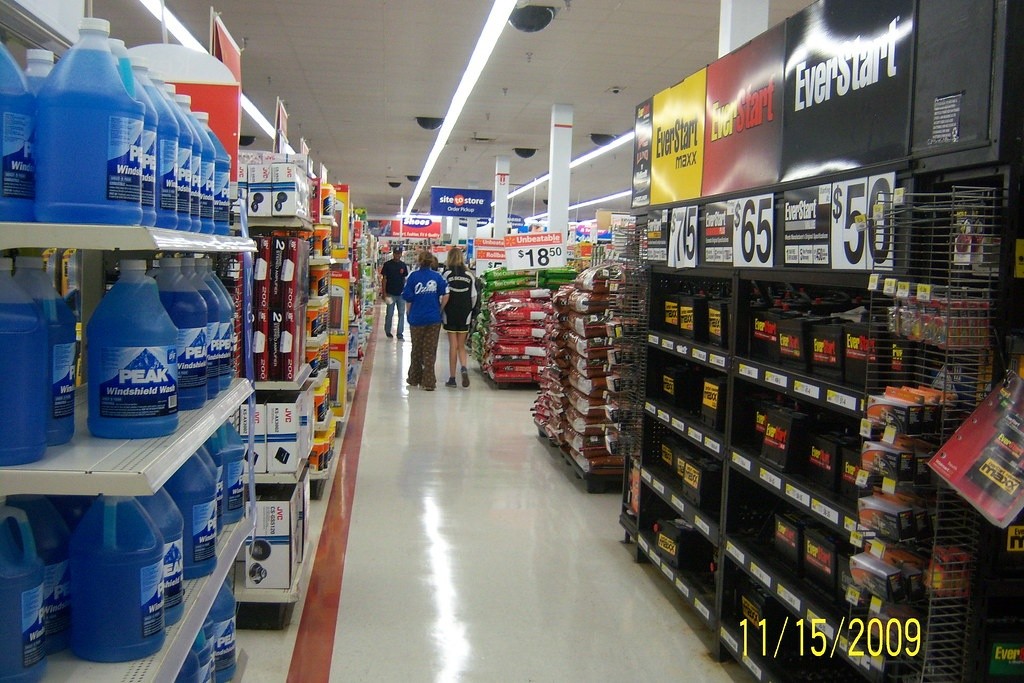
[393,246,402,253]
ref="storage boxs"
[237,164,313,586]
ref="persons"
[401,251,450,391]
[440,248,477,388]
[380,245,408,339]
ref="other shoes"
[386,332,393,337]
[406,378,417,386]
[462,371,469,387]
[445,381,456,387]
[426,386,434,391]
[397,334,403,339]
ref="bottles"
[0,16,246,682]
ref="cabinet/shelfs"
[0,217,254,683]
[310,214,335,473]
[237,216,314,604]
[616,265,1024,683]
